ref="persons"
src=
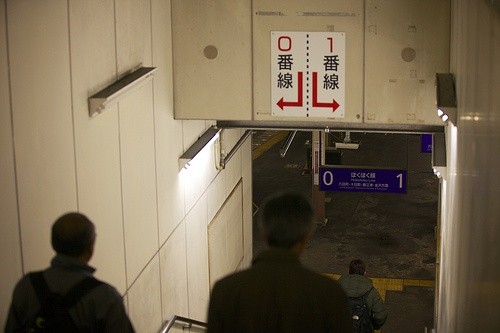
[205,190,354,333]
[3,212,136,333]
[338,259,388,333]
